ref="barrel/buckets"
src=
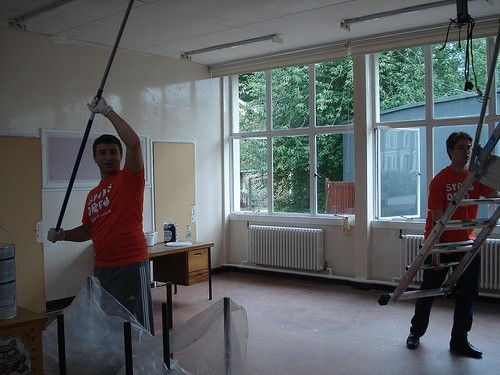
[164,223,178,244]
[0,243,17,319]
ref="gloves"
[47,227,65,244]
[86,95,113,116]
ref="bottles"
[185,225,192,242]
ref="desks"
[147,240,215,300]
[0,305,49,375]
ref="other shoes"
[407,333,420,349]
[450,340,483,356]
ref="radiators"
[399,234,500,291]
[242,225,324,272]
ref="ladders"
[378,122,500,305]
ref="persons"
[407,132,500,357]
[47,96,157,342]
